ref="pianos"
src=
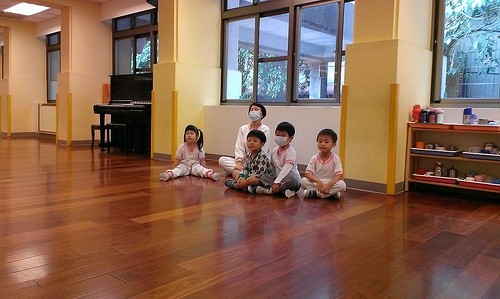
[91,73,154,158]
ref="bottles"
[411,105,444,124]
[463,107,472,124]
[434,161,442,177]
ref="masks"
[248,111,262,121]
[274,136,289,147]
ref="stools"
[91,123,127,154]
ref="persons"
[300,128,346,199]
[218,102,271,176]
[260,121,301,199]
[225,130,273,193]
[159,124,220,180]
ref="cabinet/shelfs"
[405,122,500,192]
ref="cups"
[468,146,481,153]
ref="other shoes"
[160,173,169,180]
[248,185,253,194]
[225,180,238,188]
[285,190,295,198]
[332,192,341,199]
[211,173,220,180]
[303,189,317,198]
[255,185,267,194]
